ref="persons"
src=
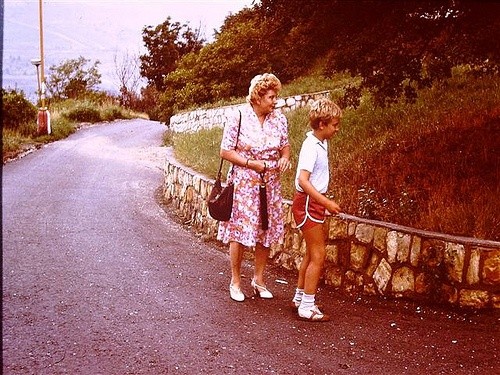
[220,72,292,302]
[289,98,342,322]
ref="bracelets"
[246,158,251,168]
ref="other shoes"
[298,306,330,321]
[291,299,298,310]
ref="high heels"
[229,282,244,301]
[251,278,273,298]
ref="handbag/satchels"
[208,176,234,221]
